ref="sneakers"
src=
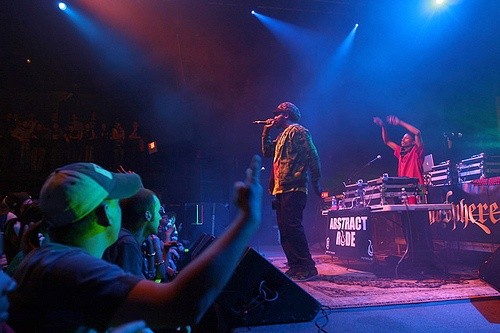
[284,266,319,282]
[407,265,431,275]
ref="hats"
[272,101,301,123]
[39,162,141,227]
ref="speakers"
[478,247,500,293]
[156,232,322,333]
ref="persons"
[159,213,169,241]
[8,153,264,333]
[261,101,323,282]
[0,108,144,162]
[373,115,432,262]
[102,189,164,277]
[0,191,182,333]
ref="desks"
[321,204,451,257]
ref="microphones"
[253,121,274,124]
[370,155,381,163]
[443,132,462,137]
